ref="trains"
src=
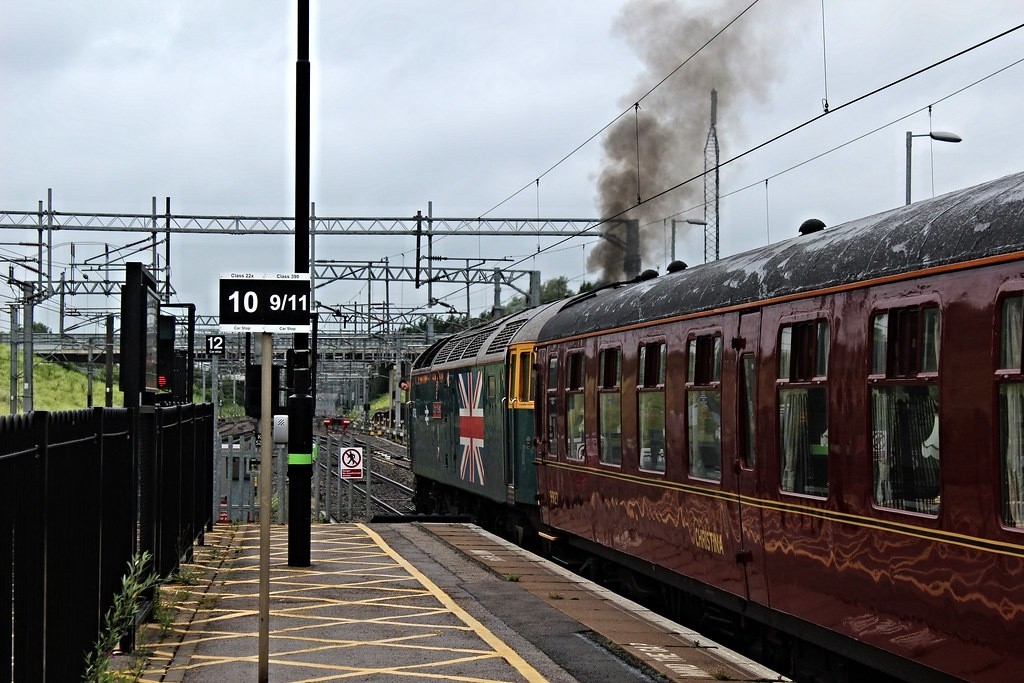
[406,171,1023,682]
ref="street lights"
[904,129,963,207]
[669,218,708,263]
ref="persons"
[399,380,411,392]
